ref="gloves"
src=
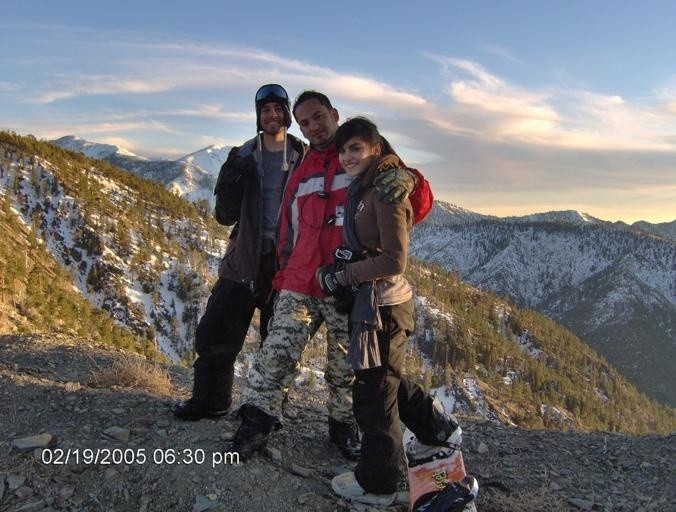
[225,145,252,175]
[316,263,349,296]
[373,167,415,204]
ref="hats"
[255,84,292,131]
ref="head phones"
[299,189,337,230]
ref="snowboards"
[402,428,479,512]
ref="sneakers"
[223,418,271,462]
[328,413,371,463]
[331,470,398,507]
[409,416,459,457]
[173,398,233,423]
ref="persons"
[223,90,436,467]
[314,115,467,510]
[168,83,310,422]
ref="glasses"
[255,84,289,101]
